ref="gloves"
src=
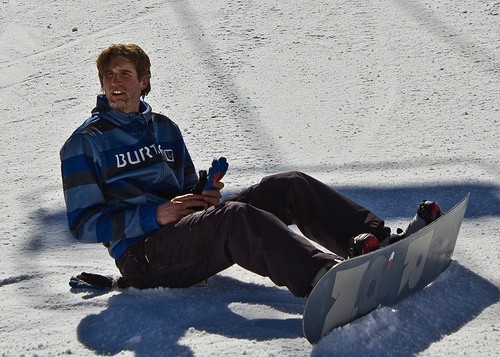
[70,272,128,291]
[190,156,229,210]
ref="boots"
[379,198,442,247]
[310,232,379,292]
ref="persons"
[60,44,444,305]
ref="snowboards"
[303,191,471,346]
